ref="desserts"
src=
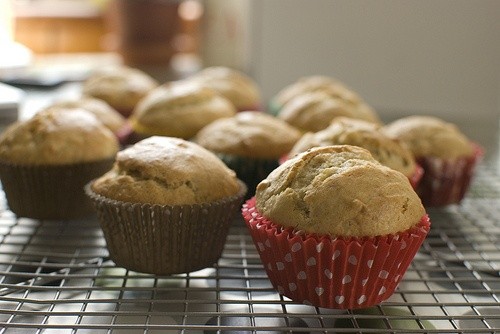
[0,66,485,311]
